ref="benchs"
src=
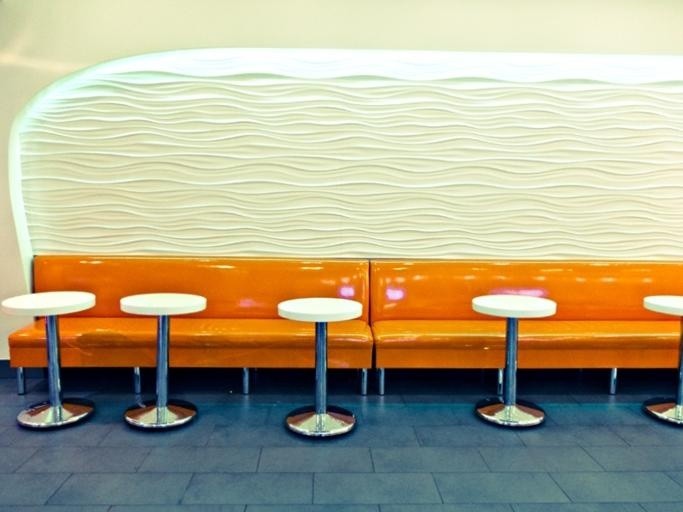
[9,255,374,403]
[368,257,683,400]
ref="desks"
[1,289,682,439]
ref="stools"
[5,292,97,431]
[643,296,683,429]
[277,297,364,439]
[120,294,207,430]
[472,295,558,431]
